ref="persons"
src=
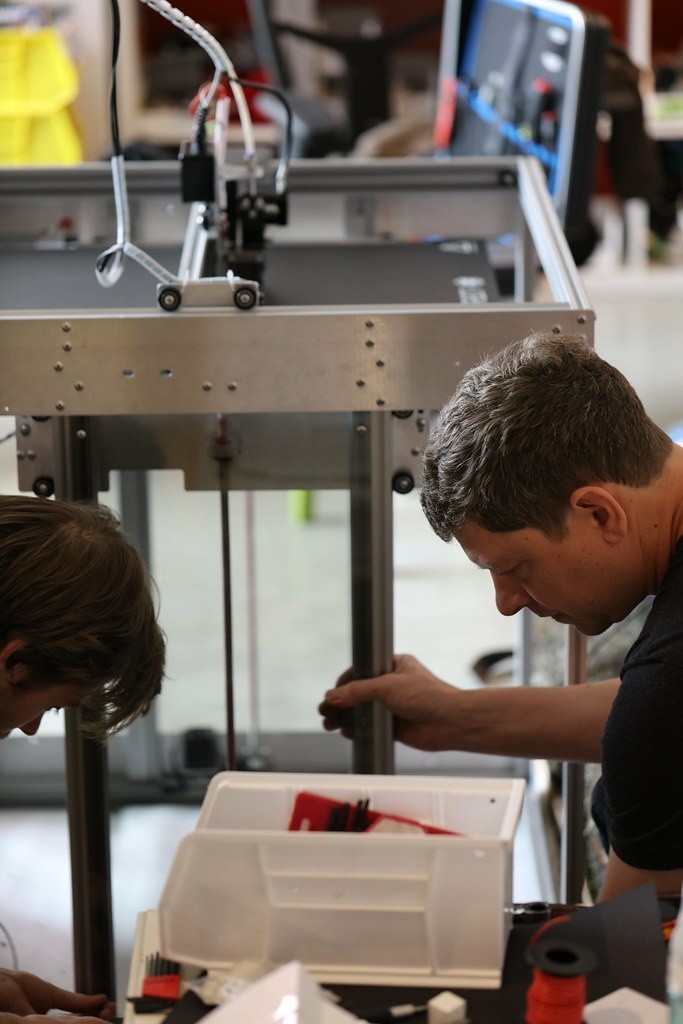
[0,494,167,1023]
[317,337,683,906]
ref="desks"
[123,897,683,1024]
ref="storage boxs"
[157,769,529,990]
